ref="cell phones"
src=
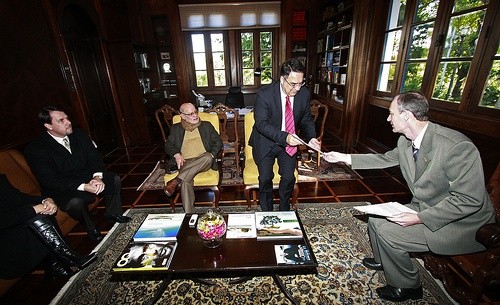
[189,214,198,225]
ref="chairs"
[155,86,328,212]
[420,163,500,305]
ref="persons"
[320,91,496,302]
[129,243,175,266]
[0,173,98,279]
[29,105,132,241]
[248,59,321,212]
[165,103,223,215]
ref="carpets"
[136,152,363,191]
[48,202,461,305]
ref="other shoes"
[164,180,177,196]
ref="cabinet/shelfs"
[312,4,355,138]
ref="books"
[255,211,304,241]
[275,244,313,265]
[112,241,179,271]
[133,213,186,241]
[314,46,346,104]
[226,214,258,239]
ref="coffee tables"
[109,210,318,305]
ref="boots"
[29,215,98,274]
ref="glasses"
[283,76,305,87]
[180,110,198,116]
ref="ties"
[285,96,298,157]
[64,138,72,149]
[412,146,419,160]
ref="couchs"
[0,144,104,296]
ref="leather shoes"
[88,228,102,240]
[376,282,423,301]
[107,216,131,223]
[362,258,384,271]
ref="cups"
[198,107,203,113]
